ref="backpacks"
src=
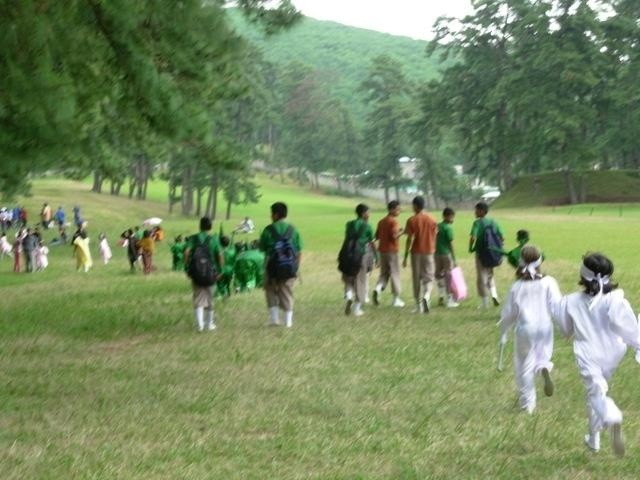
[188,236,217,286]
[337,219,369,275]
[476,218,502,268]
[265,225,298,279]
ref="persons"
[339,204,379,316]
[373,201,406,308]
[116,225,165,275]
[508,230,544,270]
[259,201,304,330]
[403,196,438,314]
[434,207,461,308]
[494,246,568,416]
[469,202,499,310]
[563,251,640,458]
[0,203,113,273]
[171,217,265,297]
[183,217,224,335]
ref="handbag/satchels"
[446,266,469,301]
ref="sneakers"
[540,367,553,396]
[198,321,215,331]
[340,290,499,316]
[611,423,625,458]
[264,319,279,327]
[585,434,599,453]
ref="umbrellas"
[143,217,163,227]
